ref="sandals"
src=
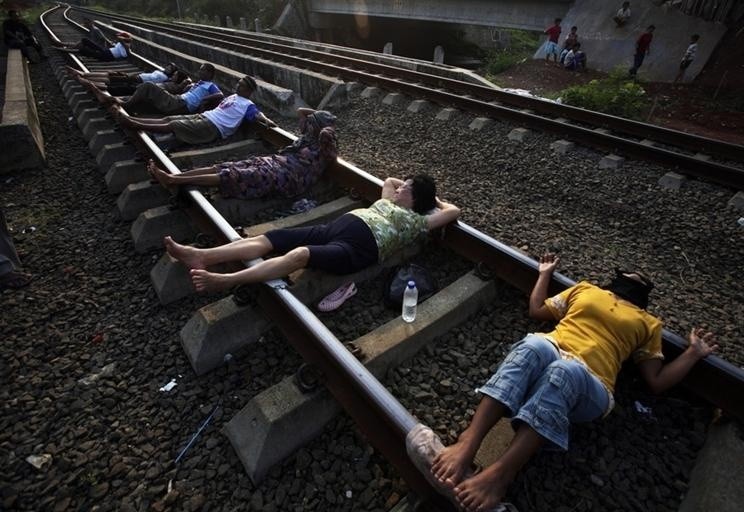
[318,281,358,312]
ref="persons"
[64,62,178,84]
[564,27,578,48]
[108,76,278,146]
[147,106,340,201]
[163,172,463,296]
[1,10,50,65]
[562,43,585,71]
[88,62,224,115]
[668,33,699,94]
[0,206,37,290]
[543,17,562,69]
[55,33,133,60]
[624,24,656,82]
[75,69,193,97]
[426,249,721,510]
[611,2,631,30]
[559,46,572,65]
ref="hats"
[116,32,132,40]
[242,75,257,91]
[307,110,337,132]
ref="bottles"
[402,280,418,324]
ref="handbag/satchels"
[383,262,441,308]
